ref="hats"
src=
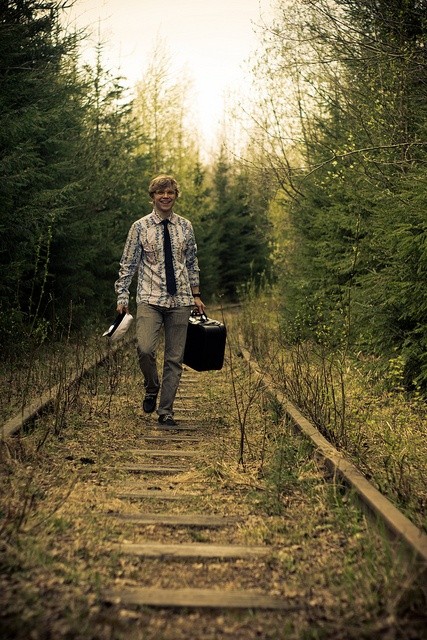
[103,311,134,342]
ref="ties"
[162,219,176,295]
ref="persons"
[115,175,206,426]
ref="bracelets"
[192,293,201,297]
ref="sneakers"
[158,414,176,425]
[143,389,158,413]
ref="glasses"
[153,190,175,195]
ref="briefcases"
[183,307,227,372]
